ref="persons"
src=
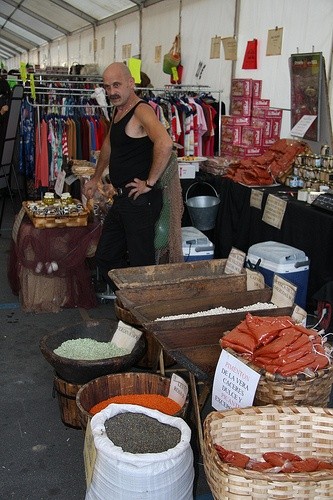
[0,78,11,115]
[82,63,173,302]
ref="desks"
[179,168,333,322]
[14,213,103,313]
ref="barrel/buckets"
[183,180,221,230]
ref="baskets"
[203,405,333,500]
[219,330,333,408]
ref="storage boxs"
[246,241,311,310]
[221,79,282,159]
[180,226,214,262]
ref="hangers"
[24,89,216,123]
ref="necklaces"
[124,97,139,112]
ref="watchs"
[145,180,153,188]
